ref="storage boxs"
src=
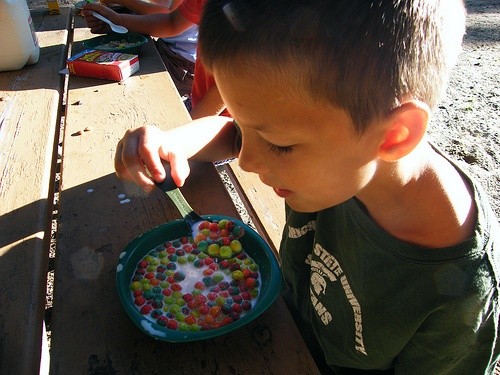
[66,48,141,83]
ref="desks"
[0,0,328,375]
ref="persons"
[81,0,232,119]
[99,0,199,98]
[114,0,500,375]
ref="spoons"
[143,158,244,259]
[88,10,128,34]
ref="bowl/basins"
[116,215,282,343]
[83,33,148,54]
